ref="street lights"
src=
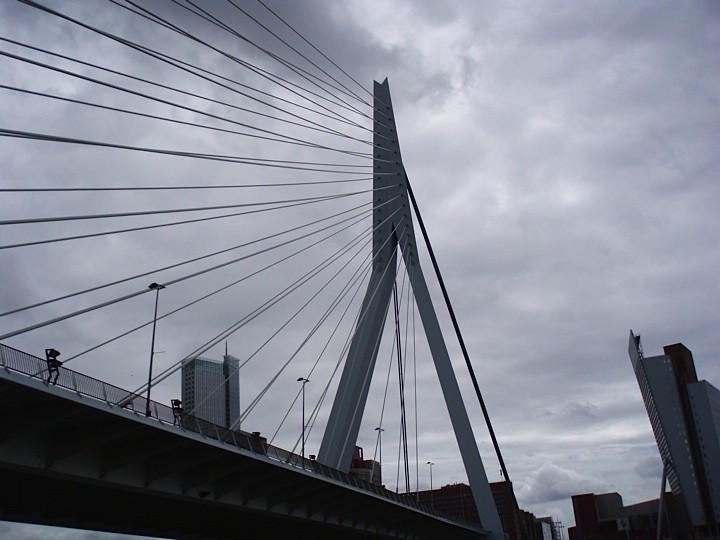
[374,427,385,483]
[145,282,166,417]
[296,378,311,470]
[426,461,436,511]
